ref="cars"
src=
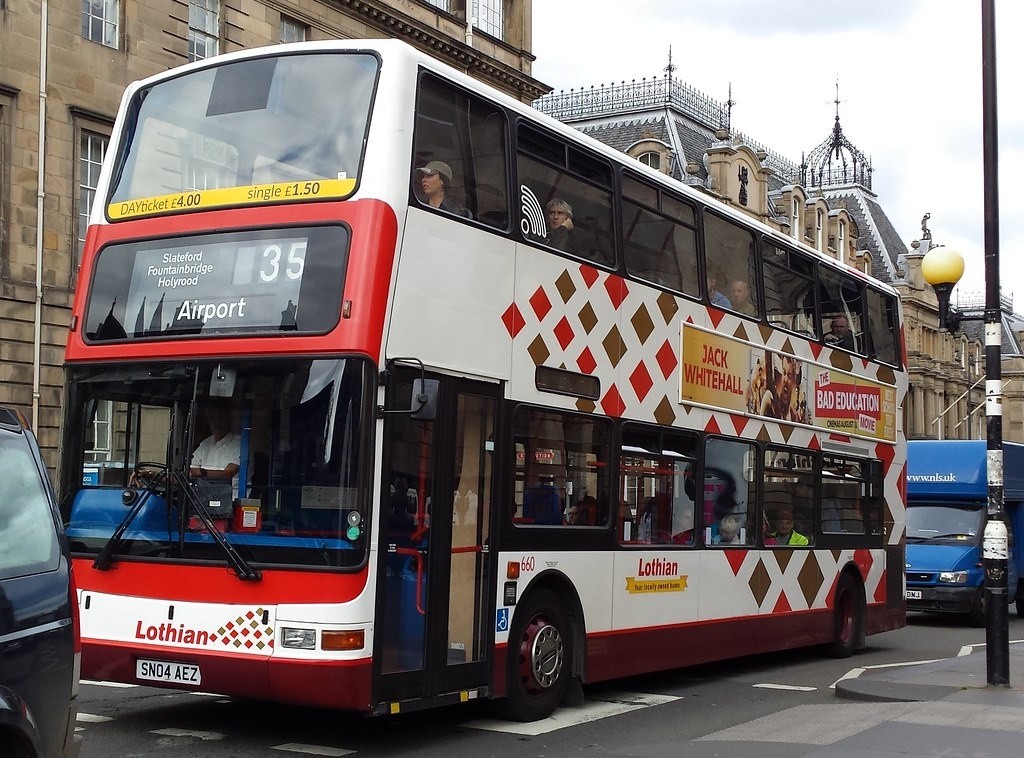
[0,404,86,758]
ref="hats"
[416,161,453,186]
[775,510,792,520]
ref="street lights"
[920,246,1011,686]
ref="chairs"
[228,407,268,458]
[768,321,863,353]
[459,207,663,285]
[748,481,882,541]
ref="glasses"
[568,512,582,516]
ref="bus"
[55,33,912,724]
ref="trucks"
[906,439,1024,625]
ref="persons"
[713,477,844,544]
[130,401,255,508]
[532,199,588,260]
[729,281,756,316]
[691,262,732,310]
[748,355,803,424]
[416,161,471,220]
[568,505,585,526]
[823,314,855,353]
